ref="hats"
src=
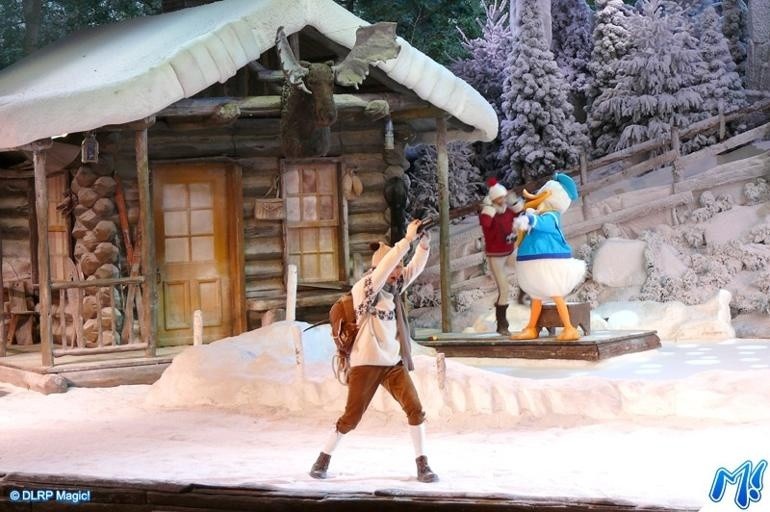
[487,178,507,201]
[371,241,404,269]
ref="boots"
[517,289,526,306]
[309,452,331,479]
[495,303,512,336]
[416,455,439,483]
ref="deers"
[274,21,401,165]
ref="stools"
[536,301,592,337]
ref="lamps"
[384,117,395,149]
[81,128,99,163]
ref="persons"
[477,177,518,339]
[311,217,442,485]
[509,173,587,341]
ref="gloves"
[406,219,431,249]
[483,196,492,205]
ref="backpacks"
[329,290,359,356]
